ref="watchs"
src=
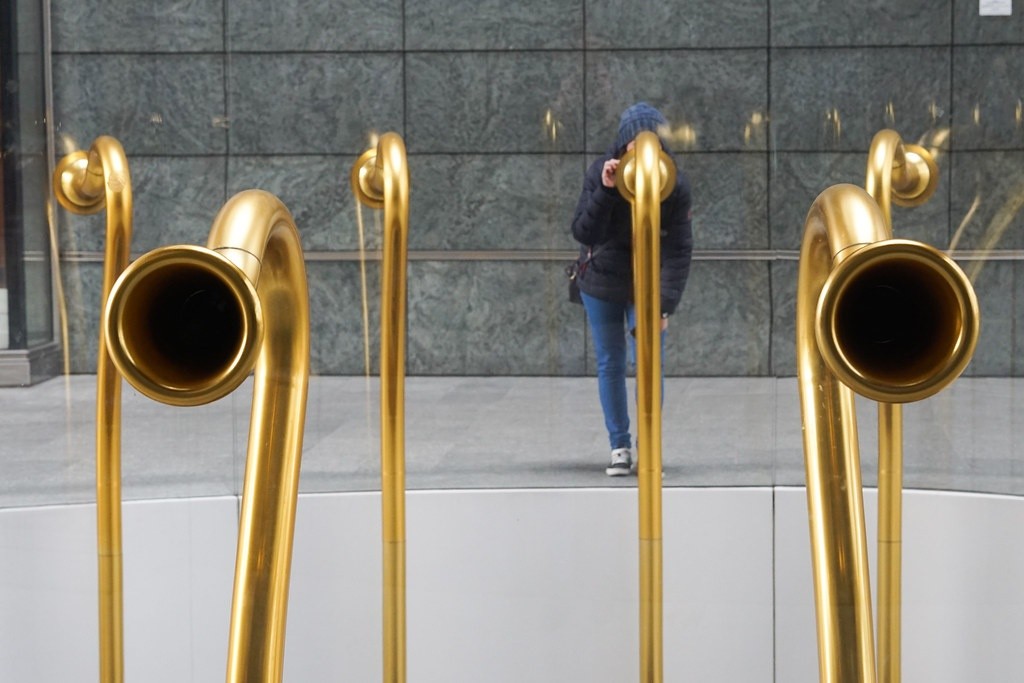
[661,312,669,319]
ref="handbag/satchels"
[568,271,585,305]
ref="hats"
[616,103,665,149]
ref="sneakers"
[607,449,631,475]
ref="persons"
[571,102,694,480]
